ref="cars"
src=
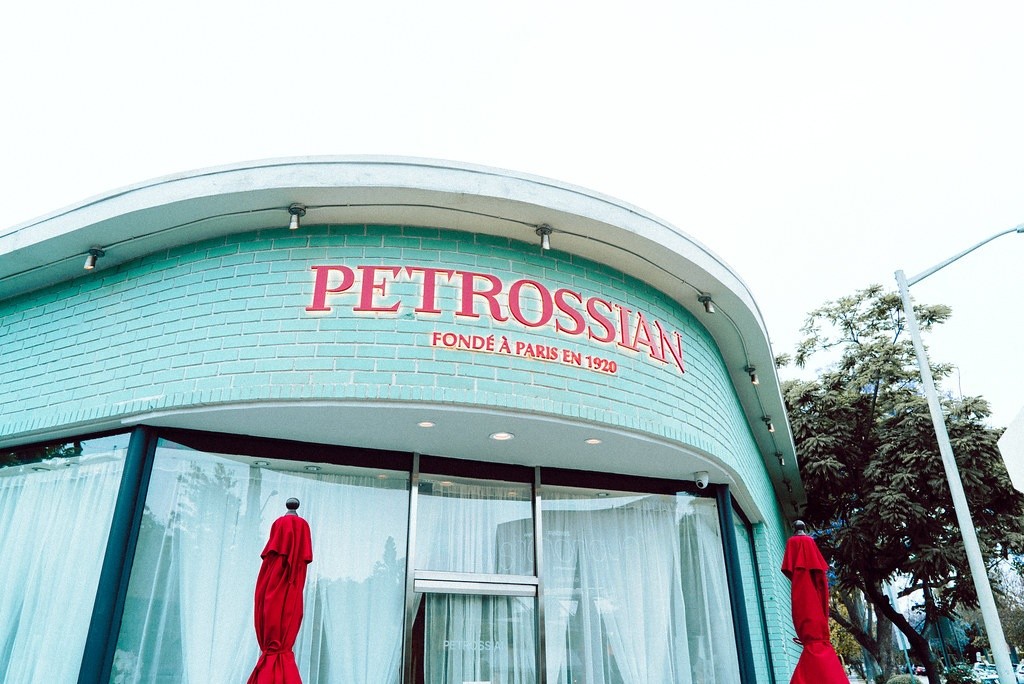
[895,657,1024,684]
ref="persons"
[845,662,852,676]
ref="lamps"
[774,450,786,467]
[744,364,759,385]
[288,202,308,231]
[697,292,716,314]
[83,243,105,270]
[762,415,775,433]
[782,478,799,514]
[535,223,554,251]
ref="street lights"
[895,220,1024,684]
